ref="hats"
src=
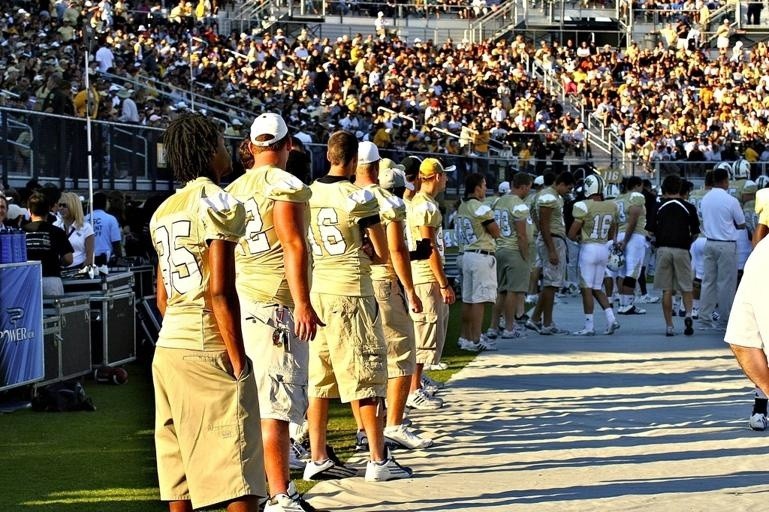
[401,155,423,173]
[498,182,509,193]
[250,112,288,147]
[418,157,456,179]
[357,141,383,165]
[534,175,544,185]
[380,168,414,190]
[378,157,406,180]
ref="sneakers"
[603,320,620,335]
[355,429,368,452]
[541,322,569,335]
[684,313,693,335]
[405,375,445,410]
[572,328,594,336]
[303,458,359,481]
[487,314,542,339]
[671,307,720,320]
[383,423,433,449]
[457,333,497,352]
[666,327,679,336]
[289,438,312,470]
[364,456,413,482]
[749,405,767,430]
[431,362,448,370]
[614,294,660,314]
[263,480,317,512]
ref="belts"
[465,250,494,255]
[707,238,736,242]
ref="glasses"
[59,202,68,208]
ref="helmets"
[607,245,625,272]
[713,161,732,180]
[732,159,750,178]
[756,175,769,189]
[584,174,603,198]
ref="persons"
[1,181,154,297]
[379,154,768,432]
[216,108,328,511]
[302,127,413,486]
[1,0,768,199]
[239,132,312,473]
[144,109,268,512]
